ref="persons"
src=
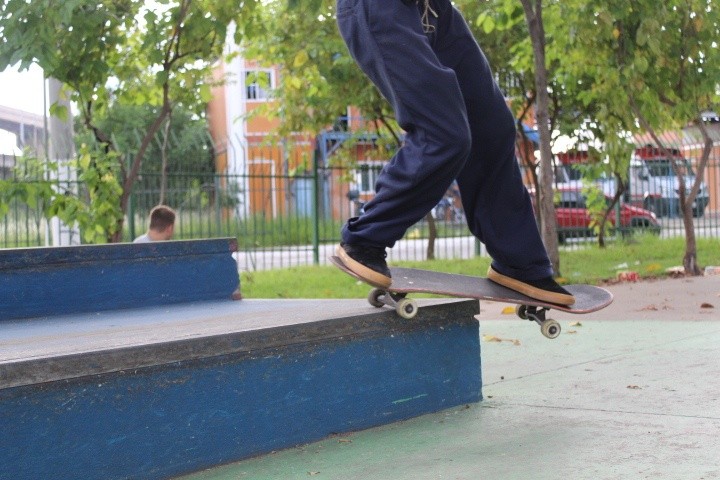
[335,0,578,306]
[132,204,175,243]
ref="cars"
[525,186,660,245]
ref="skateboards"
[330,253,611,339]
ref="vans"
[549,147,710,215]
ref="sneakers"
[338,237,394,286]
[486,256,578,307]
